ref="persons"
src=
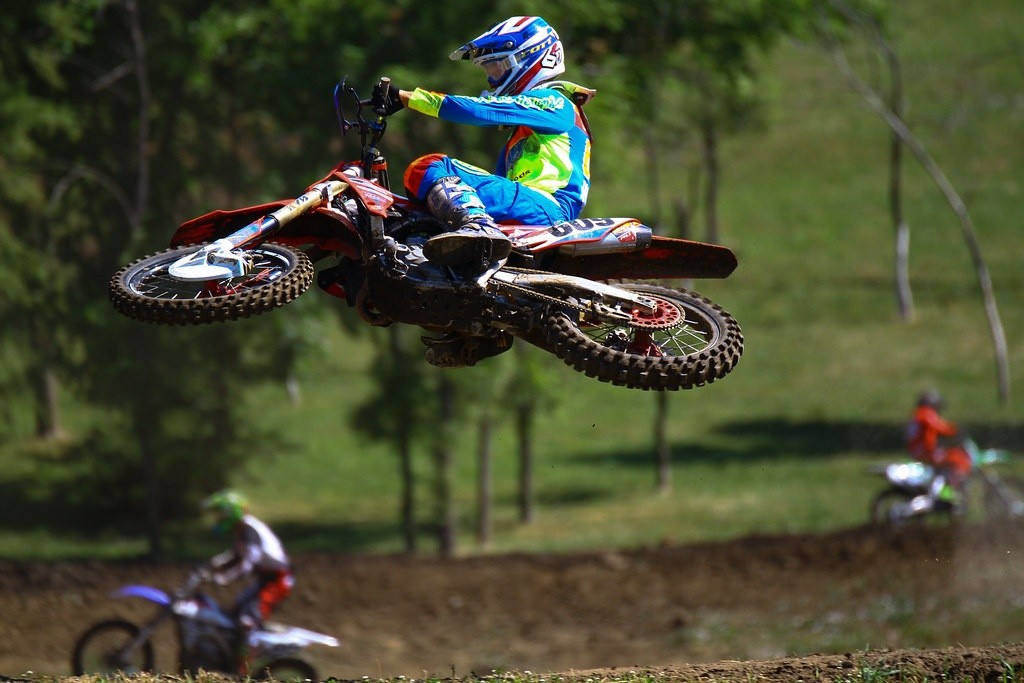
[910,381,972,506]
[197,488,298,678]
[373,16,593,370]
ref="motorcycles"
[71,570,340,683]
[865,434,1023,532]
[109,76,746,391]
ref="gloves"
[371,82,405,117]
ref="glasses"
[480,55,518,87]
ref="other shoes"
[940,485,960,506]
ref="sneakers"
[421,326,514,368]
[422,214,512,267]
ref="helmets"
[200,490,241,536]
[448,16,565,99]
[919,391,940,408]
